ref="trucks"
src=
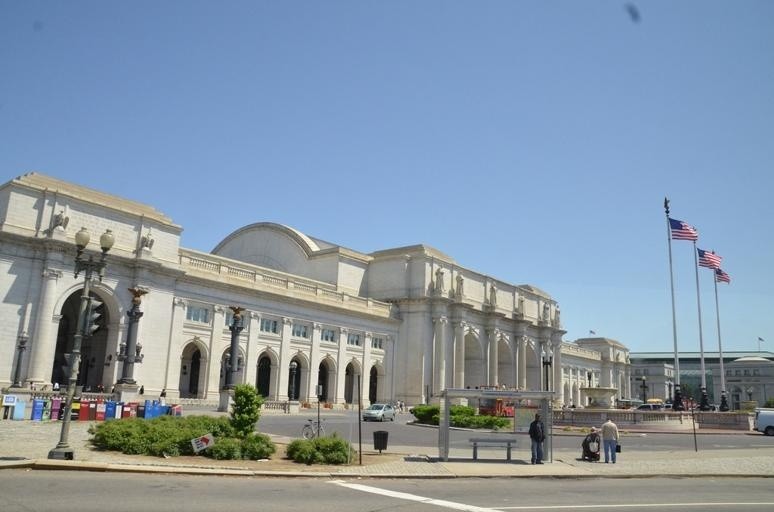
[752,407,774,437]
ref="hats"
[590,426,597,431]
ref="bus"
[477,384,533,419]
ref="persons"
[488,280,496,304]
[53,379,59,390]
[29,381,33,390]
[395,399,401,415]
[602,416,621,463]
[554,304,560,321]
[581,427,600,462]
[542,300,549,319]
[159,388,166,398]
[527,412,545,464]
[517,290,525,313]
[455,269,464,294]
[435,262,446,291]
[137,384,144,394]
[96,383,104,392]
[400,400,405,414]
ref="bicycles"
[300,417,327,442]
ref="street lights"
[747,385,752,399]
[11,331,29,388]
[640,375,649,404]
[111,286,150,404]
[47,223,114,462]
[291,360,297,401]
[587,372,593,406]
[215,305,247,414]
[688,395,697,452]
[539,349,554,392]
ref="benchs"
[467,435,518,459]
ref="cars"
[635,403,673,410]
[362,403,395,423]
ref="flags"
[696,247,723,270]
[668,218,698,242]
[589,329,595,335]
[716,268,731,285]
[758,336,765,343]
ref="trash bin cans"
[374,430,388,453]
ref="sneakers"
[604,460,616,463]
[531,461,544,464]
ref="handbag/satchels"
[615,444,621,452]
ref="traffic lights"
[85,297,104,338]
[62,351,74,380]
